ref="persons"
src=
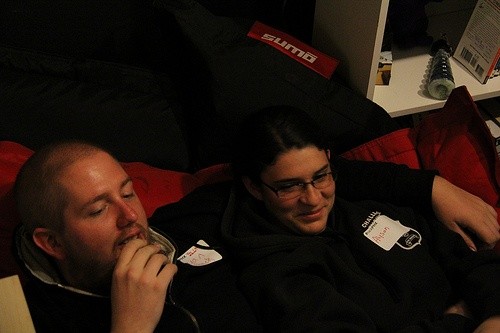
[220,123,499,333]
[0,139,499,333]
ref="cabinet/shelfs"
[313,0,500,119]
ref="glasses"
[258,148,338,202]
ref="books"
[451,0,500,84]
